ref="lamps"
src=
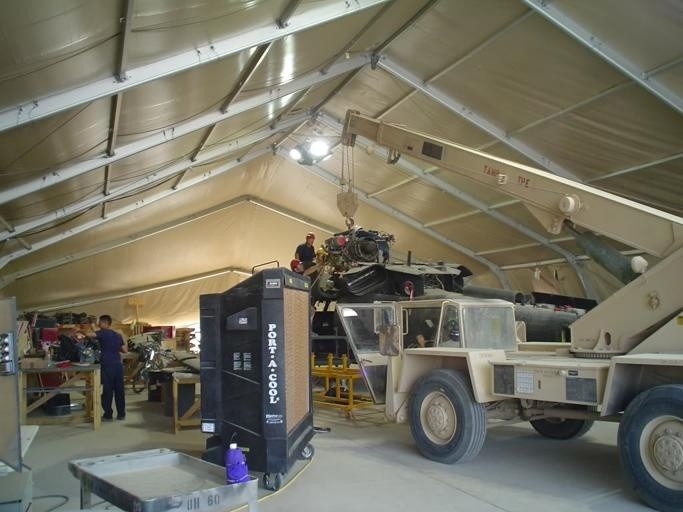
[291,139,332,166]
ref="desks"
[161,373,201,433]
[18,363,101,429]
[67,446,259,512]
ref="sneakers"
[101,416,124,422]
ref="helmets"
[291,259,303,269]
[306,233,315,239]
[315,248,325,254]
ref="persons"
[399,281,426,348]
[291,232,347,336]
[72,315,129,422]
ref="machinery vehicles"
[336,110,683,512]
